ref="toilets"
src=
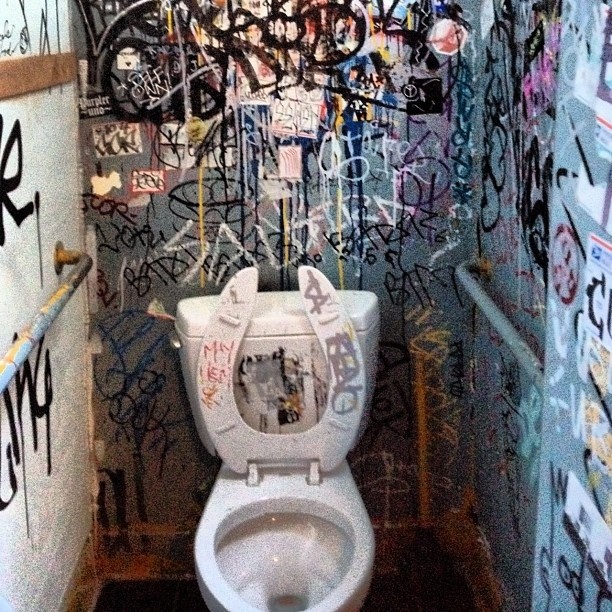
[175,266,381,612]
